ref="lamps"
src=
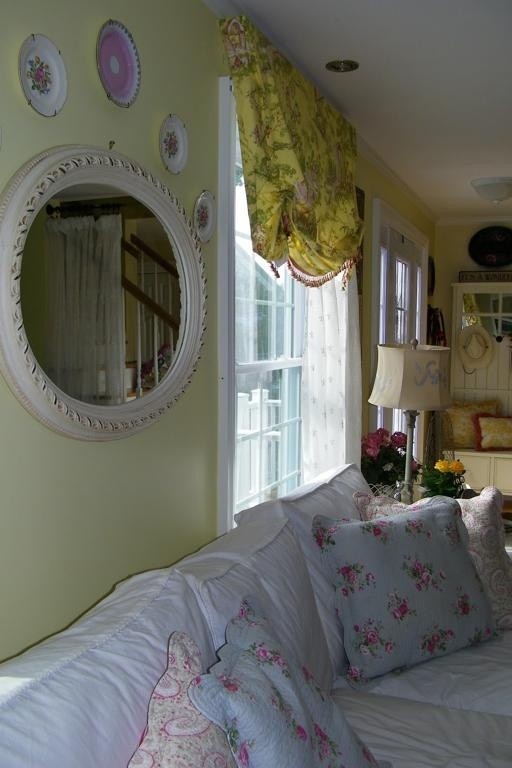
[471,177,512,208]
[368,339,455,505]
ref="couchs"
[1,463,512,768]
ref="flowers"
[361,427,466,498]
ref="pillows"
[442,398,512,451]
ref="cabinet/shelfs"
[450,282,512,416]
[67,368,137,397]
[442,448,512,495]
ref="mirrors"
[461,293,512,336]
[1,144,208,442]
